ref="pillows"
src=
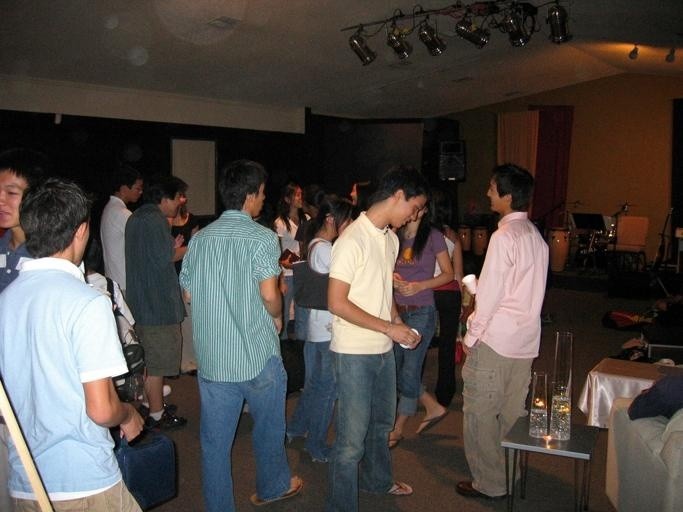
[660,408,683,441]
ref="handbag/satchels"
[109,431,183,511]
[290,238,334,311]
[277,248,303,270]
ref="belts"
[394,304,419,316]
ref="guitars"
[653,208,673,272]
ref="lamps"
[341,0,572,65]
[666,46,676,62]
[627,43,639,59]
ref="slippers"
[384,431,404,450]
[414,407,451,435]
[385,480,413,497]
[249,474,302,506]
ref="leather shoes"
[455,480,506,502]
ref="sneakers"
[159,384,171,397]
[136,400,179,415]
[143,412,187,430]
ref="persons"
[456,163,551,499]
[0,159,464,512]
[628,374,683,421]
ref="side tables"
[500,416,600,512]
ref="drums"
[457,225,471,251]
[548,227,569,271]
[473,227,487,255]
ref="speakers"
[439,141,465,181]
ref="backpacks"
[85,274,147,402]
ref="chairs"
[564,316,644,388]
[603,396,683,512]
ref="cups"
[461,274,477,294]
[399,329,418,349]
